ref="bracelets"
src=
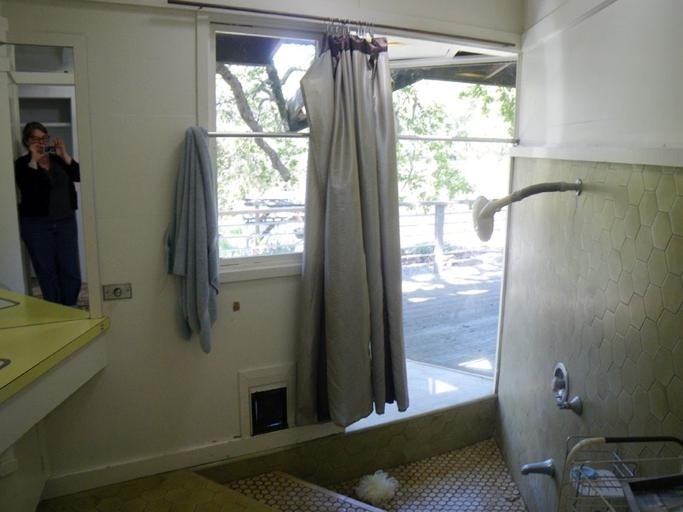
[29,161,37,167]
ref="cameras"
[45,146,56,152]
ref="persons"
[15,121,81,306]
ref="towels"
[169,127,220,354]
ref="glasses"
[27,135,50,142]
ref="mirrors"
[0,31,102,329]
